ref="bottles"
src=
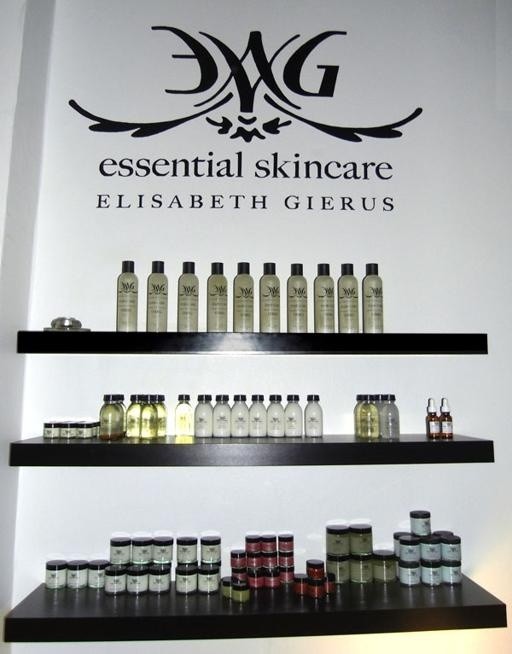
[115,259,383,335]
[212,394,231,438]
[438,398,452,440]
[267,394,285,438]
[229,394,250,438]
[194,394,213,439]
[303,395,323,438]
[99,393,168,442]
[426,398,441,439]
[352,394,400,440]
[284,394,303,438]
[248,395,267,438]
[175,393,194,438]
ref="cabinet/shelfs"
[5,329,507,642]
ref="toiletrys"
[258,261,281,332]
[206,260,228,332]
[146,259,169,332]
[178,260,199,333]
[286,262,308,333]
[361,262,384,333]
[233,261,255,333]
[337,262,360,334]
[313,262,336,333]
[116,260,138,331]
